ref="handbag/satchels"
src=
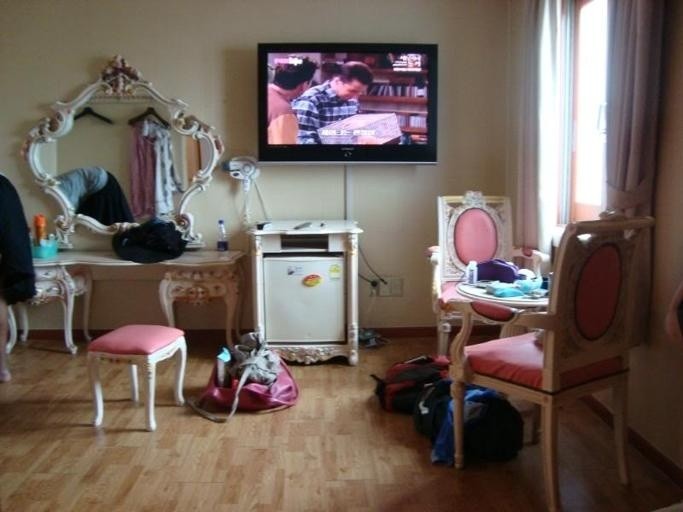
[197,350,300,416]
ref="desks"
[453,271,552,400]
[1,248,249,359]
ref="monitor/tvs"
[257,42,439,165]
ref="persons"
[267,58,319,146]
[290,60,374,145]
[54,164,134,226]
[0,174,37,383]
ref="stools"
[80,322,192,436]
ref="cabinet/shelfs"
[244,217,365,370]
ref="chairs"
[422,190,552,362]
[441,206,656,511]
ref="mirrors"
[18,54,227,253]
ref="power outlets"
[368,276,380,297]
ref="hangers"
[125,97,171,132]
[68,98,113,126]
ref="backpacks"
[373,348,524,472]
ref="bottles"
[466,261,477,284]
[31,212,45,244]
[214,219,228,257]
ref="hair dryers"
[220,159,256,193]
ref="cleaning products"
[33,214,48,245]
